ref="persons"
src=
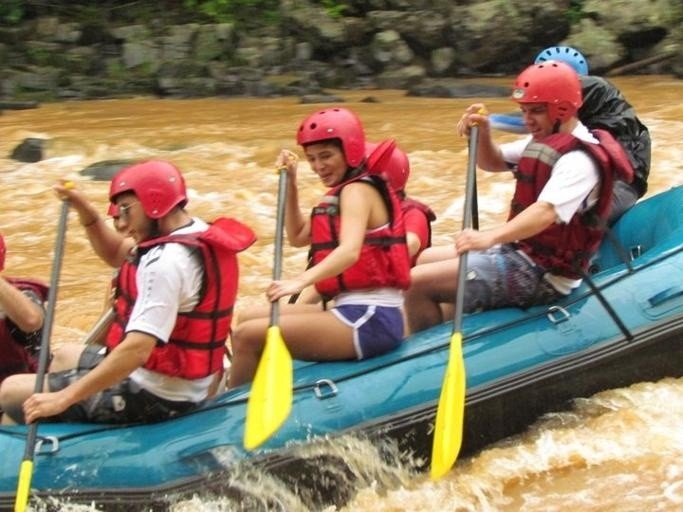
[1,160,257,426]
[105,205,130,308]
[467,44,651,245]
[0,236,55,419]
[404,59,632,339]
[367,139,436,266]
[224,107,409,391]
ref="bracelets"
[79,214,100,228]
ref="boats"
[485,110,525,133]
[0,184,682,506]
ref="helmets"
[365,142,409,192]
[296,108,365,168]
[108,160,188,220]
[511,60,583,124]
[536,45,588,76]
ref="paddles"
[243,155,294,452]
[430,123,479,480]
